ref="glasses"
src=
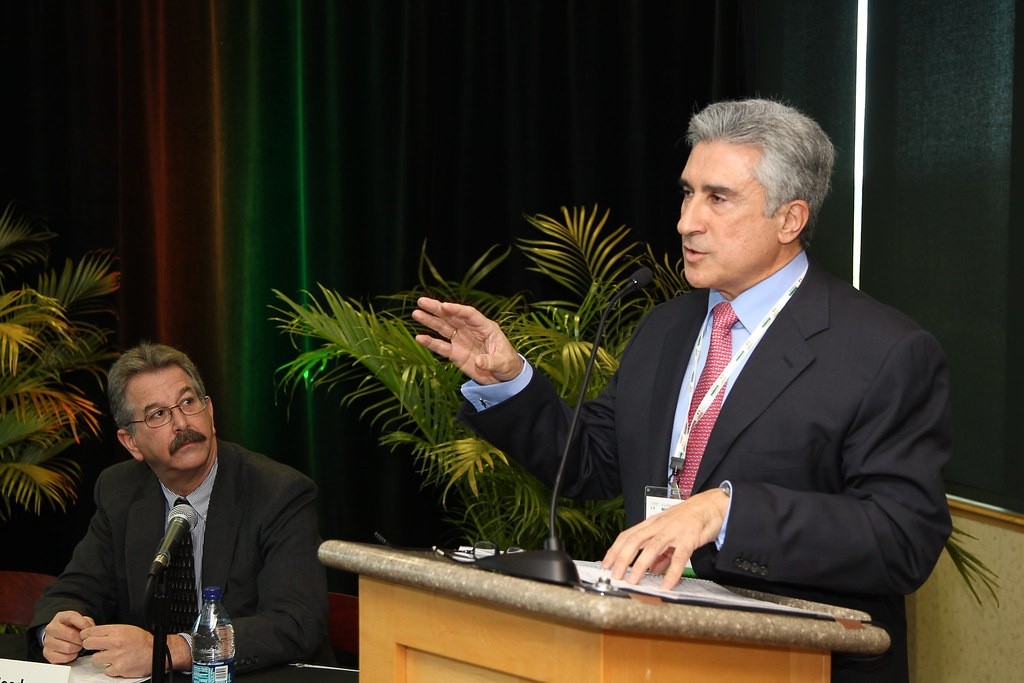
[119,396,206,429]
[431,540,527,565]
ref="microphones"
[476,268,654,586]
[147,505,197,578]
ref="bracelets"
[722,487,730,497]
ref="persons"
[409,99,955,683]
[27,344,327,678]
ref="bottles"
[191,586,237,683]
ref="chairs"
[327,591,359,655]
[0,571,58,626]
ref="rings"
[449,329,457,339]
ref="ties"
[647,302,739,575]
[148,500,198,632]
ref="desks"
[0,634,359,683]
